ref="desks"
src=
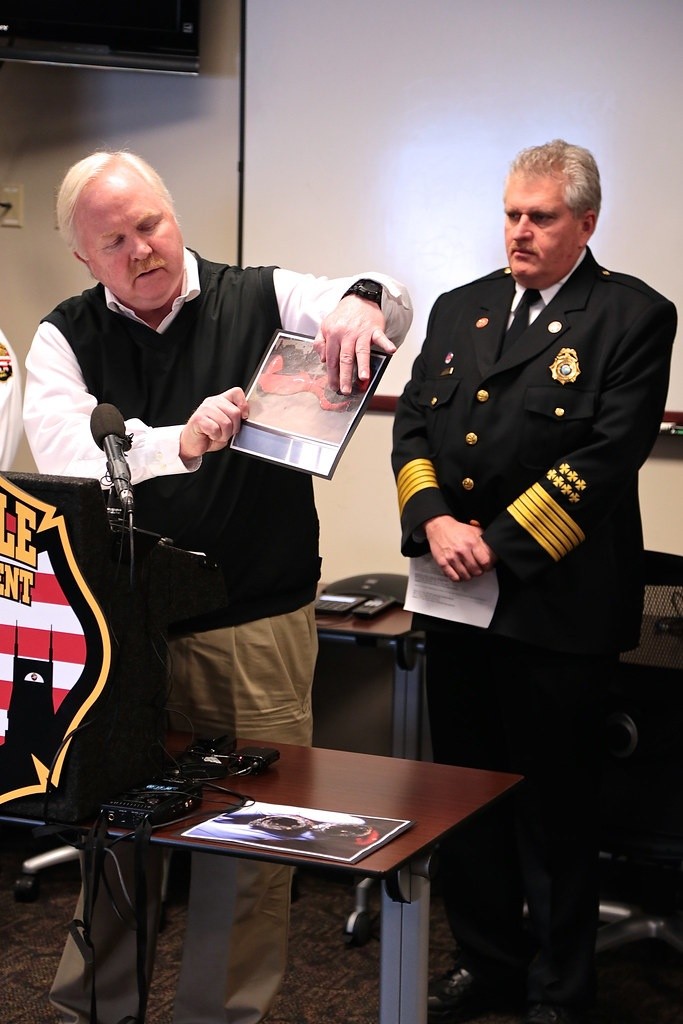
[312,583,431,761]
[0,737,525,1024]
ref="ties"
[501,288,543,355]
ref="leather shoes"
[527,977,597,1024]
[427,965,528,1024]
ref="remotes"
[352,598,395,619]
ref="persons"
[391,138,681,1024]
[22,150,414,1024]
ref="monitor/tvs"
[0,0,200,77]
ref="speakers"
[0,477,173,822]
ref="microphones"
[90,403,134,514]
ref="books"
[179,801,412,865]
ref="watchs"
[340,279,382,311]
